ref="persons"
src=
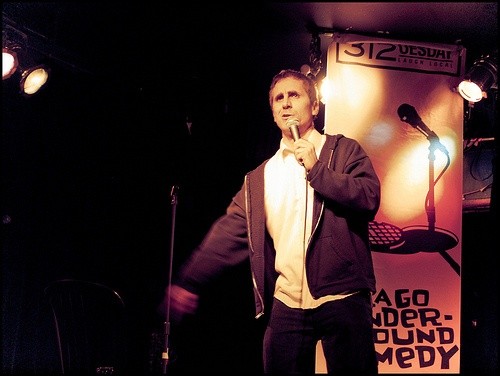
[165,69,381,376]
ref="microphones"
[286,118,305,165]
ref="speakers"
[0,280,147,376]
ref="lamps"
[0,16,51,100]
[451,54,500,109]
